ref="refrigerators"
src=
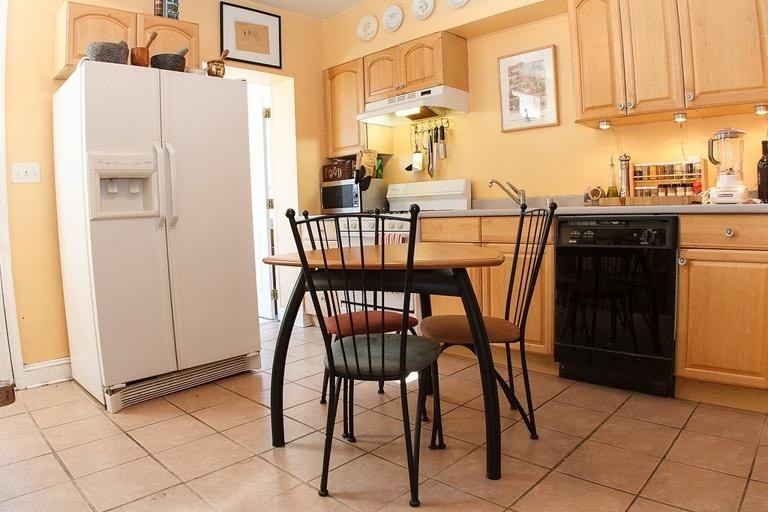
[52,59,260,413]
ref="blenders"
[708,129,745,203]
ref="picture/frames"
[219,1,281,67]
[497,44,558,133]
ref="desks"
[263,244,501,480]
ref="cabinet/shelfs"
[418,217,558,375]
[569,0,767,130]
[53,2,200,78]
[323,32,469,160]
[675,214,766,413]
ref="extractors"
[355,84,469,128]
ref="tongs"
[426,130,434,177]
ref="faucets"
[489,179,526,208]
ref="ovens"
[554,213,676,399]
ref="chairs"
[419,201,556,448]
[302,210,417,403]
[284,205,445,508]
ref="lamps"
[599,122,611,131]
[755,105,767,116]
[675,114,687,121]
[396,107,422,120]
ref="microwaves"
[320,178,385,215]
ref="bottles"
[757,140,768,206]
[173,1,178,19]
[154,0,163,16]
[168,0,173,16]
[634,162,702,197]
[607,163,617,197]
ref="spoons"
[406,130,413,171]
